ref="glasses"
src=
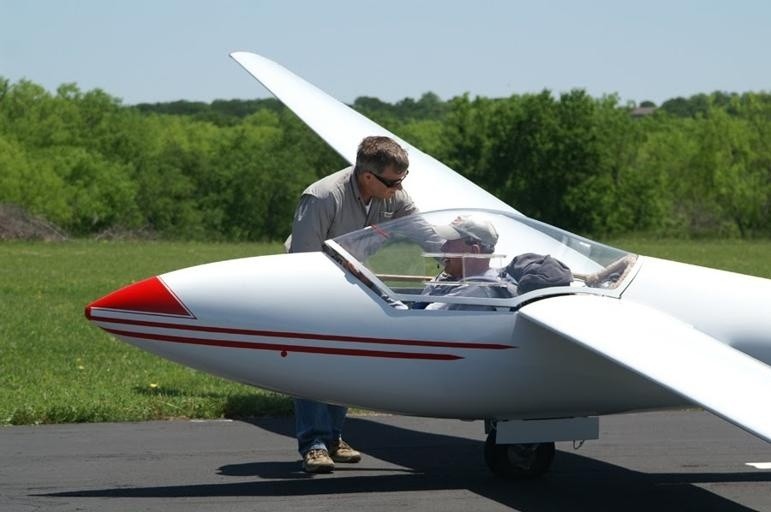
[370,170,408,188]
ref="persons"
[278,136,442,476]
[380,211,516,310]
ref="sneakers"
[304,448,334,474]
[329,439,361,463]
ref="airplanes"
[80,48,771,482]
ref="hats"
[431,214,499,247]
